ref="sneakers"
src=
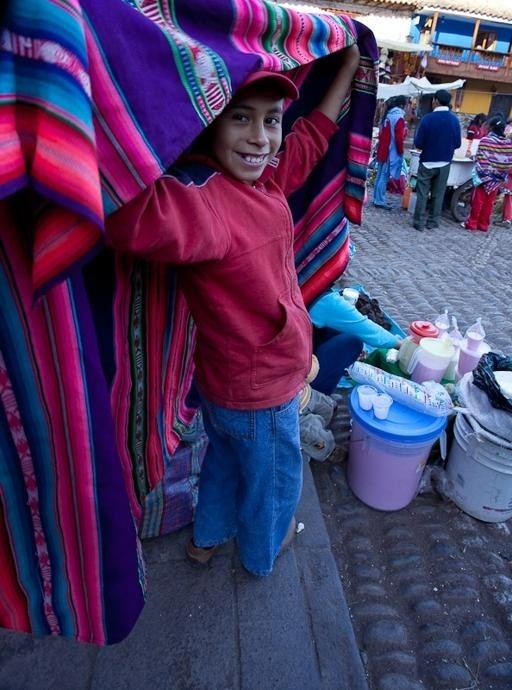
[186,514,296,574]
[414,221,437,230]
[494,220,510,227]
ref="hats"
[237,69,300,98]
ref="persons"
[299,385,350,463]
[371,95,410,210]
[104,43,361,578]
[408,89,461,231]
[460,110,512,232]
[306,287,404,396]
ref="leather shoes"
[371,200,393,210]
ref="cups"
[436,323,449,337]
[407,336,454,387]
[372,393,393,420]
[357,385,378,411]
[398,321,440,375]
[467,332,483,351]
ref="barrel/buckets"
[348,381,443,512]
[446,368,512,521]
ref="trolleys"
[408,149,475,223]
